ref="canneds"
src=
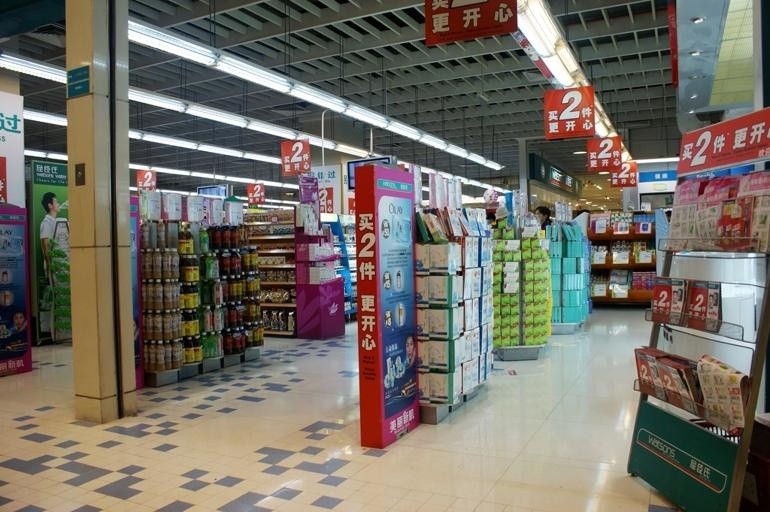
[632,271,656,289]
[243,214,269,221]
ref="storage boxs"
[415,202,494,425]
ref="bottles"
[140,219,179,248]
[209,222,240,248]
[179,337,201,363]
[153,249,162,279]
[217,332,224,356]
[164,340,173,370]
[212,248,241,279]
[176,308,182,338]
[155,279,164,309]
[225,327,245,354]
[238,222,244,248]
[154,310,162,340]
[241,270,261,296]
[200,254,206,279]
[172,339,181,369]
[149,340,158,371]
[172,249,180,279]
[142,280,147,309]
[140,250,145,279]
[161,249,172,278]
[202,280,210,303]
[213,278,223,305]
[208,332,219,358]
[143,340,150,372]
[176,279,180,309]
[243,296,262,321]
[206,253,211,280]
[199,228,210,254]
[147,279,155,309]
[201,306,224,331]
[181,310,200,336]
[242,246,258,270]
[156,341,166,371]
[163,279,172,309]
[222,301,244,327]
[171,309,178,338]
[146,310,154,340]
[179,222,194,254]
[244,222,249,247]
[222,275,242,300]
[142,310,146,339]
[145,249,153,279]
[180,283,198,309]
[164,309,172,339]
[171,279,177,309]
[181,255,200,282]
[202,333,209,357]
[245,321,265,346]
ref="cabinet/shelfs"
[587,210,671,308]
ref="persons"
[8,311,27,357]
[533,206,551,228]
[404,335,418,369]
[39,192,63,332]
